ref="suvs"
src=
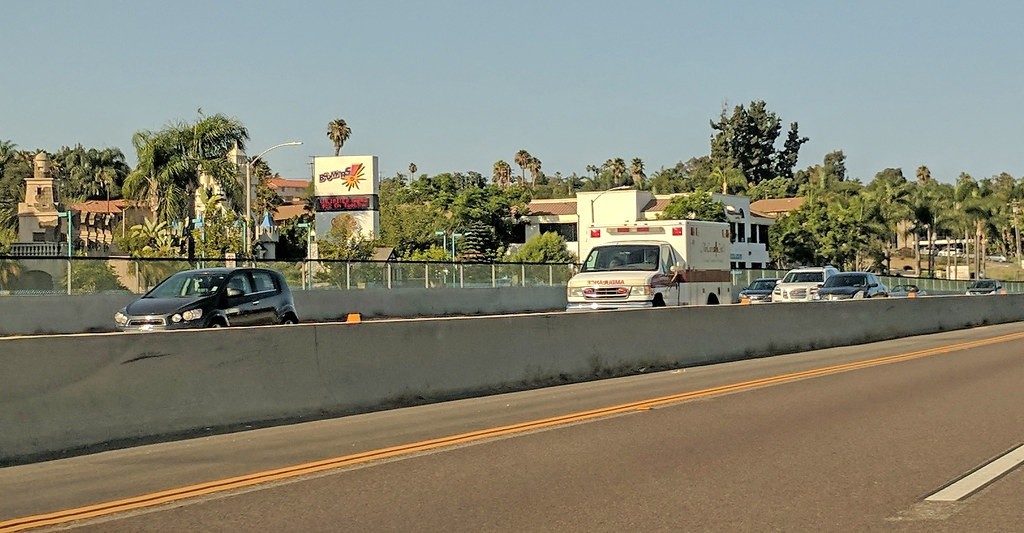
[771,265,840,301]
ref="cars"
[738,278,783,303]
[888,284,926,297]
[965,279,1007,296]
[115,266,299,333]
[812,272,888,301]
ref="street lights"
[246,141,306,261]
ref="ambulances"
[566,219,733,312]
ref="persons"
[797,274,807,282]
[196,277,219,294]
[610,258,619,269]
[644,251,659,271]
